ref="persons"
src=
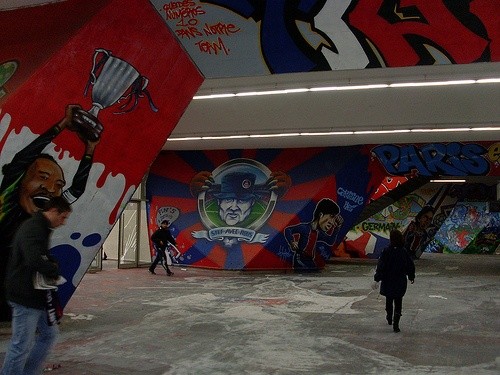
[374,230,416,332]
[149,220,178,276]
[0,196,72,375]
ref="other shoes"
[149,269,156,275]
[168,272,174,276]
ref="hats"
[161,220,168,225]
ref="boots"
[385,306,392,325]
[393,310,402,332]
